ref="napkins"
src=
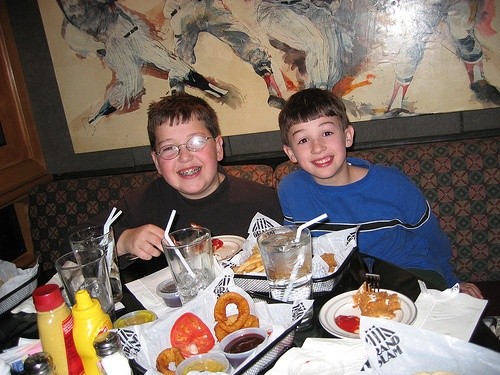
[122,257,221,317]
[413,288,489,342]
[11,260,84,314]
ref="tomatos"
[212,238,223,251]
[171,312,215,358]
[334,315,360,332]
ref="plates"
[318,287,418,339]
[210,235,245,261]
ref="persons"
[115,93,283,261]
[276,88,483,299]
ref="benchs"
[30,131,500,319]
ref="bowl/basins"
[176,352,230,375]
[219,327,268,369]
[114,310,158,329]
[155,278,182,307]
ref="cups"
[257,224,313,304]
[161,227,216,306]
[69,225,123,303]
[54,247,116,322]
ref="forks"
[363,257,380,293]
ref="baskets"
[232,230,358,293]
[0,271,40,315]
[128,289,304,375]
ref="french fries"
[233,244,266,274]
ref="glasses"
[156,135,215,160]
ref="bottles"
[93,331,133,375]
[23,352,58,375]
[32,284,86,375]
[71,289,114,375]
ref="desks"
[0,253,500,375]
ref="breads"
[352,282,402,319]
